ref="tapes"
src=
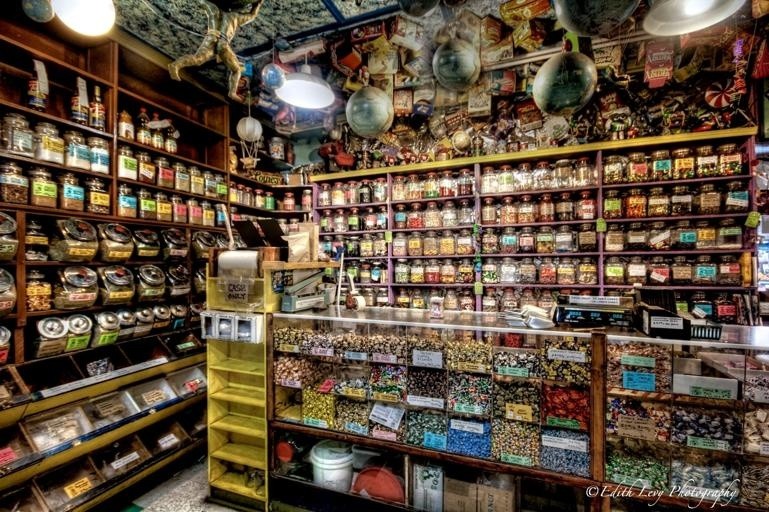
[352,296,366,312]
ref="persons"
[168,0,263,102]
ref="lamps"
[530,35,601,112]
[639,0,749,38]
[552,1,640,39]
[431,0,484,92]
[334,2,394,137]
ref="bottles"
[89,84,107,133]
[89,136,110,175]
[117,109,134,143]
[35,122,64,165]
[4,112,35,158]
[71,81,89,127]
[64,131,92,171]
[151,113,164,150]
[165,118,178,154]
[0,143,750,365]
[27,64,48,113]
[136,106,151,147]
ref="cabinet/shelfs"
[306,114,758,316]
[0,0,336,512]
[273,299,601,510]
[602,325,769,512]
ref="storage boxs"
[442,466,478,512]
[477,474,525,512]
[350,441,382,471]
[411,460,447,511]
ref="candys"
[691,77,755,132]
[274,151,769,508]
